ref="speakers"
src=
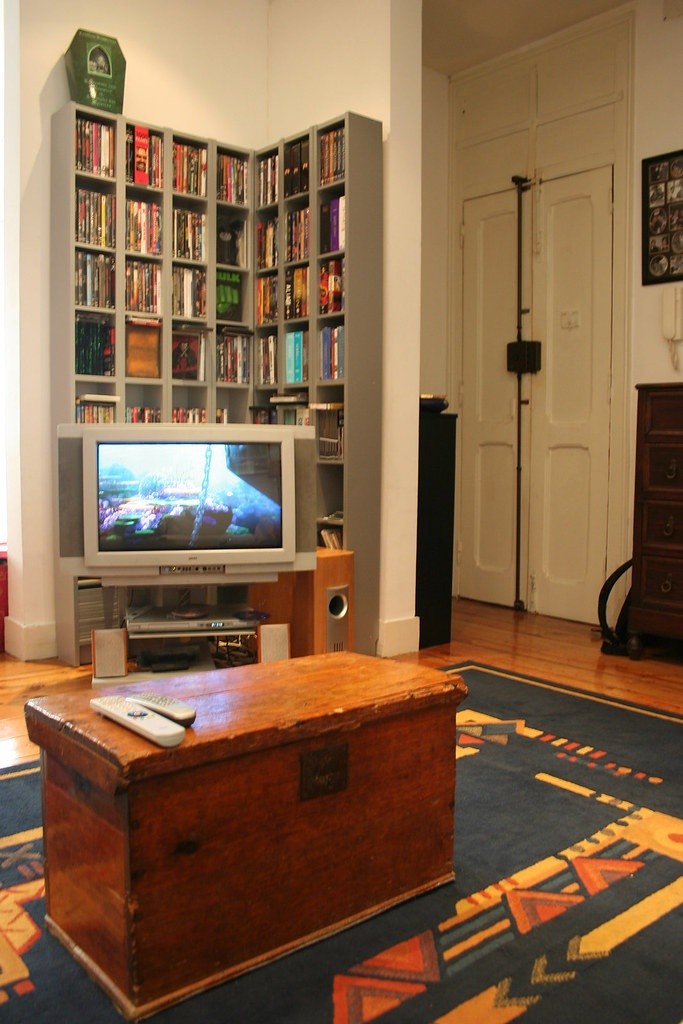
[244,547,355,658]
[256,622,292,663]
[90,628,128,678]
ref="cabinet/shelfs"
[415,413,458,649]
[629,382,682,660]
[91,571,278,691]
[51,100,384,668]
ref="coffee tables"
[24,650,468,1020]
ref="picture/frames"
[642,150,683,286]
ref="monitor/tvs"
[57,424,318,585]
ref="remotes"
[126,692,196,727]
[89,696,186,747]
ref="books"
[75,117,345,456]
[321,528,344,549]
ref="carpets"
[1,659,683,1024]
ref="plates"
[649,160,683,277]
